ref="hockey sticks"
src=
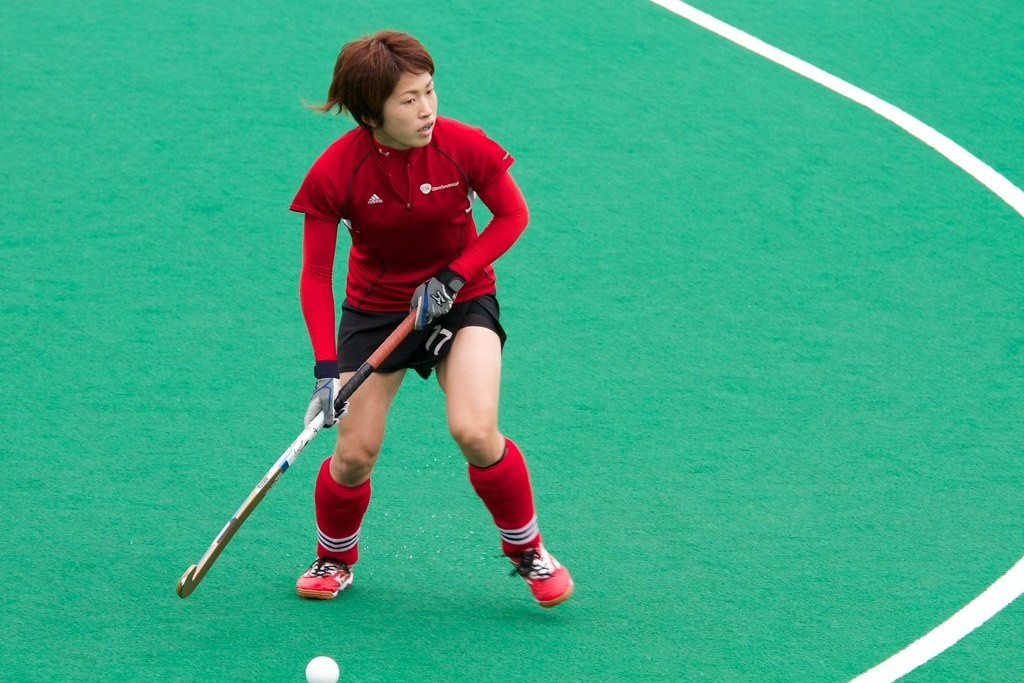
[174,302,423,600]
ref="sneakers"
[294,552,353,600]
[494,536,573,609]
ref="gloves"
[304,360,349,429]
[409,267,466,331]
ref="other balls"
[304,654,341,683]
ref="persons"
[287,31,575,608]
